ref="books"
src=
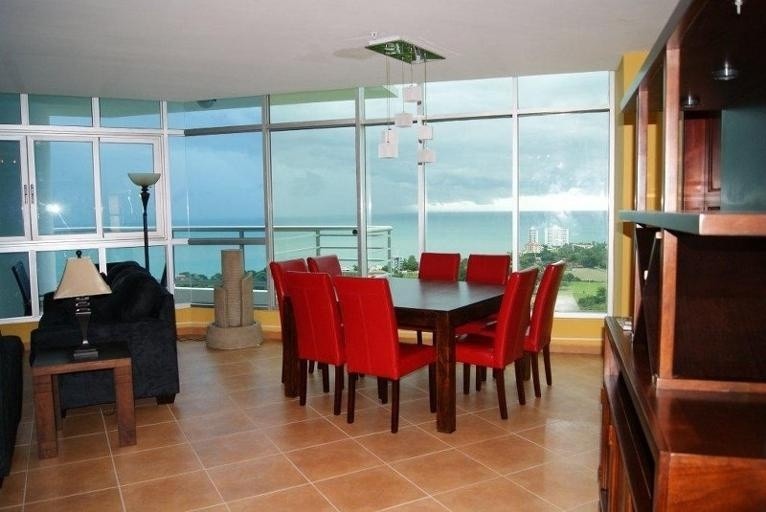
[616,314,633,332]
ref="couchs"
[0,332,24,495]
[28,259,180,408]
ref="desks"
[27,343,140,460]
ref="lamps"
[363,35,446,167]
[51,249,117,360]
[125,170,160,267]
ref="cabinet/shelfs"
[615,0,766,403]
[593,312,766,512]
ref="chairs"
[269,256,343,385]
[418,252,461,282]
[453,268,539,421]
[285,271,349,415]
[468,261,563,399]
[335,276,439,435]
[467,254,511,286]
